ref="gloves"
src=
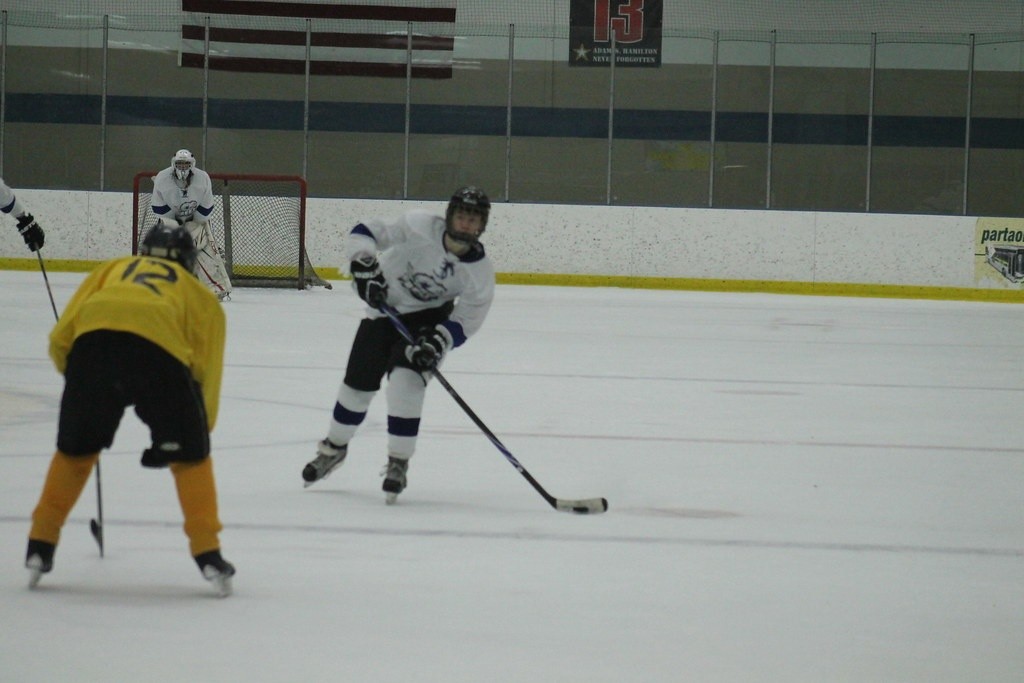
[141,440,170,468]
[402,325,447,371]
[16,212,45,252]
[352,252,388,307]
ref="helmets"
[446,184,490,243]
[141,218,197,274]
[171,148,196,181]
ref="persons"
[302,181,496,506]
[24,217,237,597]
[151,148,234,302]
[1,182,45,253]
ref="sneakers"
[25,539,56,589]
[195,548,237,598]
[303,438,347,488]
[380,456,408,504]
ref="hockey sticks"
[199,258,228,300]
[375,300,611,518]
[33,244,64,325]
[85,456,106,560]
